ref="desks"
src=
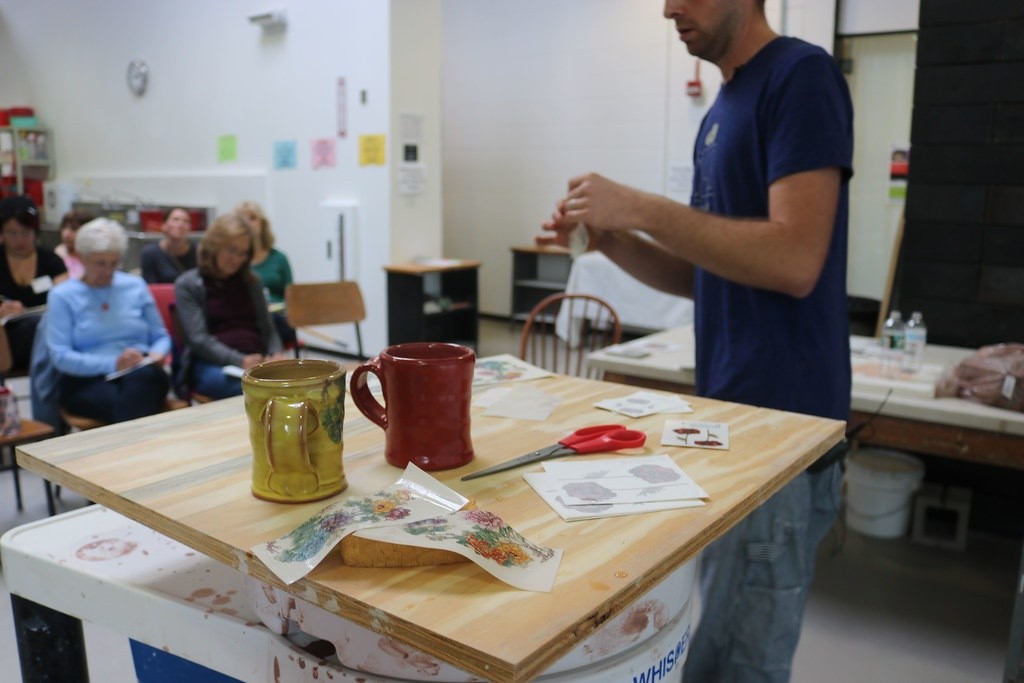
[585,323,1024,470]
[39,224,204,276]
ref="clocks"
[127,60,149,96]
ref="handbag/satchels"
[29,314,64,421]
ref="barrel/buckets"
[843,449,925,538]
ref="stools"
[909,486,972,551]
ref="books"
[223,365,245,378]
[105,357,155,382]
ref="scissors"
[461,424,647,481]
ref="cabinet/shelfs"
[509,245,573,330]
[384,258,483,358]
[0,126,57,227]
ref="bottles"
[904,312,927,372]
[0,388,19,437]
[881,311,903,373]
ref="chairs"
[0,281,365,518]
[519,294,621,381]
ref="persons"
[141,207,201,283]
[0,196,69,387]
[536,0,853,683]
[53,212,98,279]
[175,214,283,400]
[46,218,172,425]
[233,201,296,346]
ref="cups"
[241,359,347,504]
[350,342,476,471]
